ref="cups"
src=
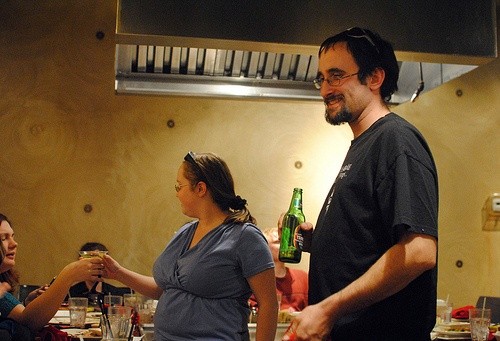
[77,251,108,262]
[68,296,88,327]
[104,293,140,339]
[437,301,453,324]
[468,309,491,341]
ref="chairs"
[476,296,500,324]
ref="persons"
[276,28,440,340]
[0,213,124,341]
[76,151,311,341]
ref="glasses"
[184,151,203,178]
[347,26,379,52]
[174,183,192,192]
[313,67,374,90]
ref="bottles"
[278,187,306,263]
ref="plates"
[61,328,146,341]
[434,321,471,337]
[49,310,102,324]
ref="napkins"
[451,304,477,318]
